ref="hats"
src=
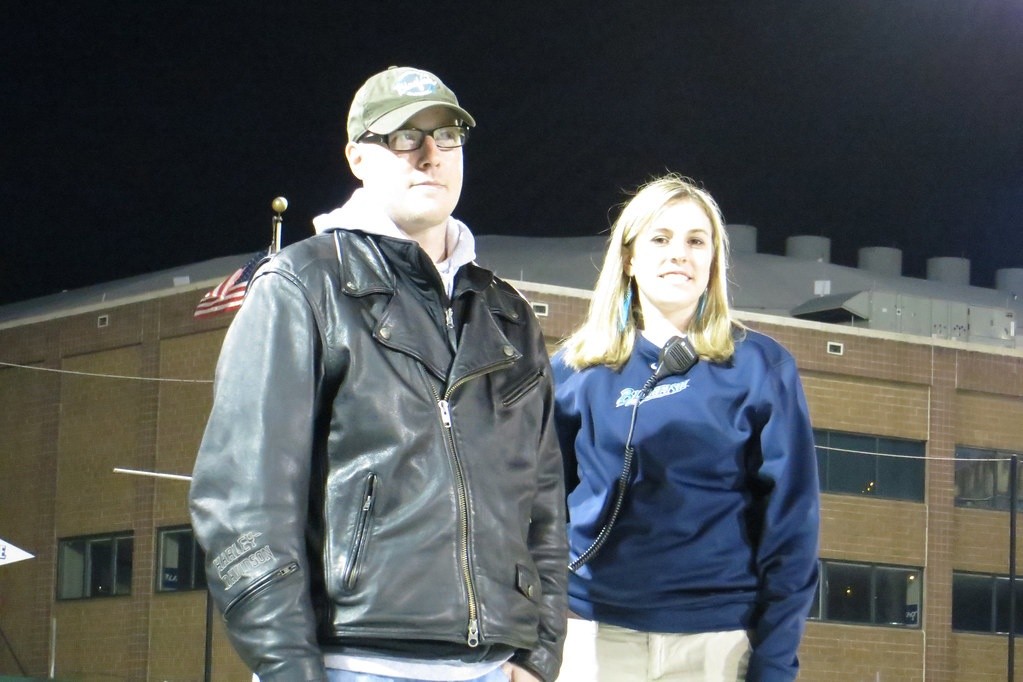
[347,65,476,142]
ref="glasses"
[358,125,469,151]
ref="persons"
[185,66,572,682]
[543,173,822,682]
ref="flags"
[192,245,271,320]
